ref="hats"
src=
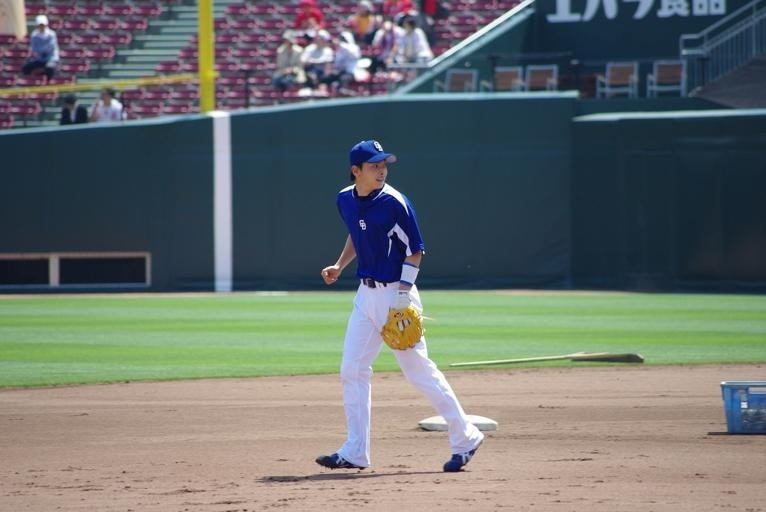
[349,140,397,165]
[35,15,49,26]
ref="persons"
[85,86,124,124]
[314,139,484,475]
[270,1,448,99]
[20,15,60,82]
[58,92,88,126]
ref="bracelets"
[399,264,419,290]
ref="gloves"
[389,290,412,332]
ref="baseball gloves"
[383,309,423,350]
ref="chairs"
[510,64,560,94]
[594,62,639,101]
[644,59,687,100]
[431,66,480,95]
[476,64,524,92]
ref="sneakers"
[443,441,483,471]
[316,453,365,470]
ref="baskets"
[720,381,766,434]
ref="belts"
[363,278,386,288]
